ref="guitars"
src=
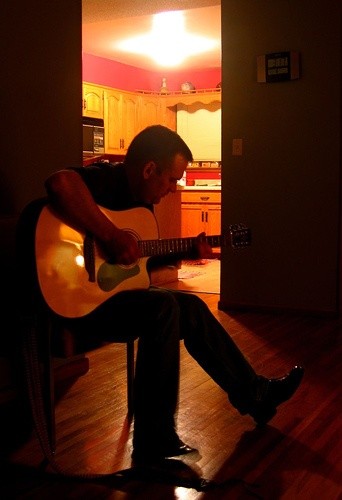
[18,196,252,322]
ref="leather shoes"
[250,365,304,425]
[132,440,201,467]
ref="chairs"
[13,198,135,453]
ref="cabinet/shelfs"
[82,150,221,286]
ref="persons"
[43,126,305,465]
